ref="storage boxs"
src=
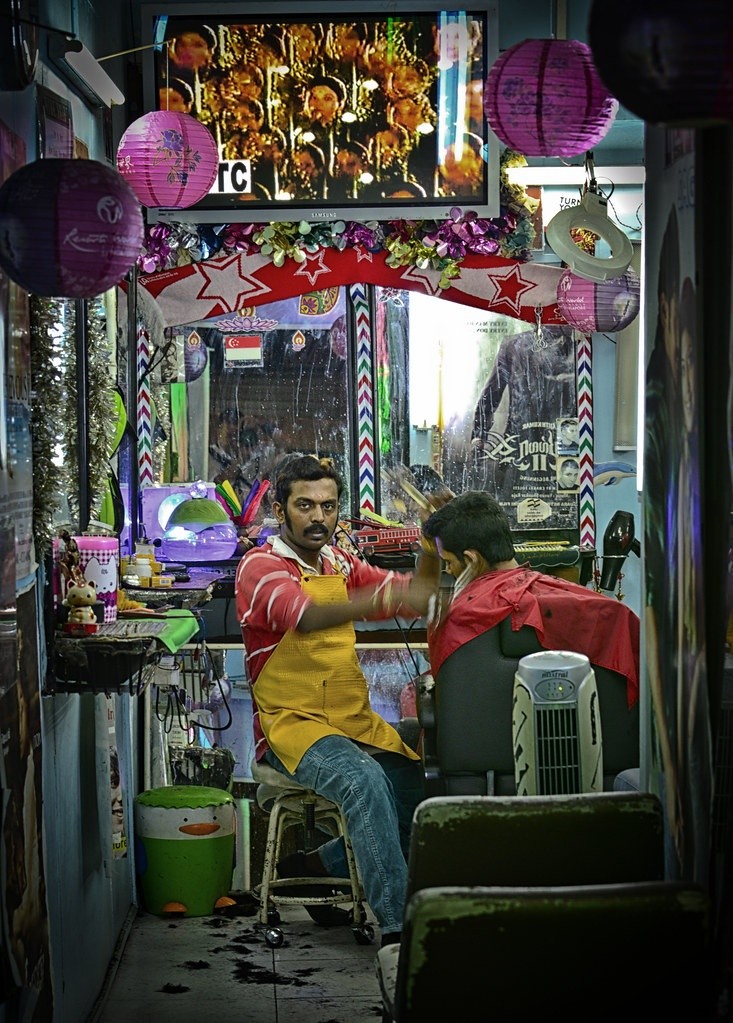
[52,535,120,625]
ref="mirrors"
[179,314,352,540]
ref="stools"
[133,783,237,919]
[250,758,374,947]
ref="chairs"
[415,592,638,795]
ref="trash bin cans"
[136,784,238,919]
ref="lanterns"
[182,329,208,381]
[118,109,219,212]
[329,314,348,363]
[0,158,145,300]
[557,260,639,334]
[483,39,619,157]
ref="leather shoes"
[276,850,350,929]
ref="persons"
[557,421,580,452]
[641,208,707,880]
[236,456,460,948]
[558,459,580,492]
[422,491,520,590]
[209,410,290,506]
[392,461,457,525]
